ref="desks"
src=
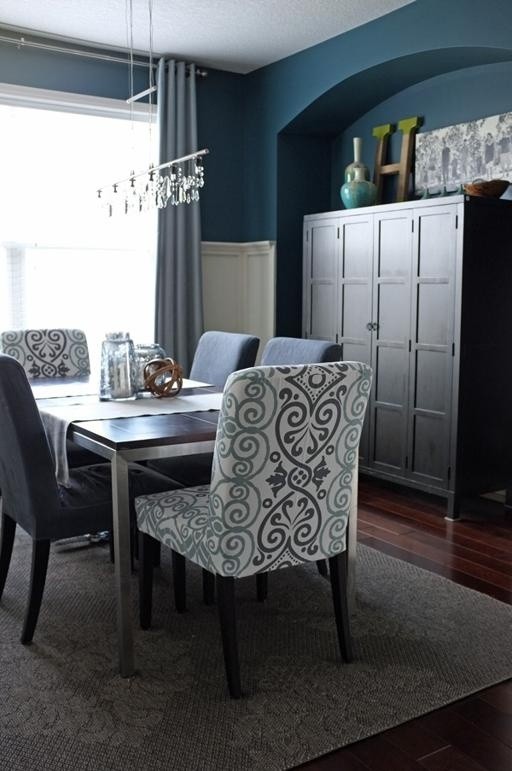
[24,369,242,684]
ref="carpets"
[0,526,512,771]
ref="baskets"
[464,178,510,199]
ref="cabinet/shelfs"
[301,193,512,520]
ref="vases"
[341,167,379,208]
[345,137,369,182]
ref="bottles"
[135,342,166,391]
[99,331,139,401]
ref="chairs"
[261,336,344,368]
[0,353,185,647]
[186,330,262,391]
[144,359,373,697]
[2,329,95,379]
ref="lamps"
[91,1,208,221]
